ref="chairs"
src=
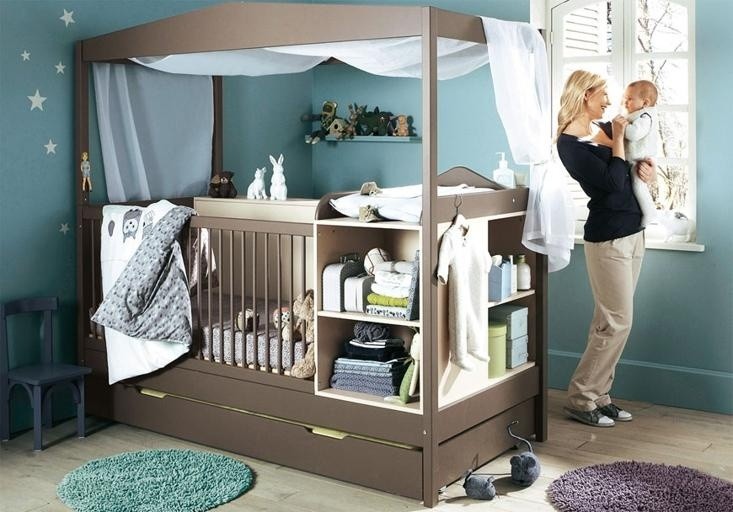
[0,293,94,453]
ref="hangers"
[444,192,475,239]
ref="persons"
[555,69,660,427]
[623,80,660,229]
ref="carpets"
[545,459,733,511]
[56,448,254,511]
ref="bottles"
[507,254,517,294]
[486,320,506,378]
[515,252,531,291]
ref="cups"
[235,313,259,333]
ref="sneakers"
[563,403,616,427]
[599,402,634,422]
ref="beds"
[69,2,551,509]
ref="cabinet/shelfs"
[311,166,540,417]
